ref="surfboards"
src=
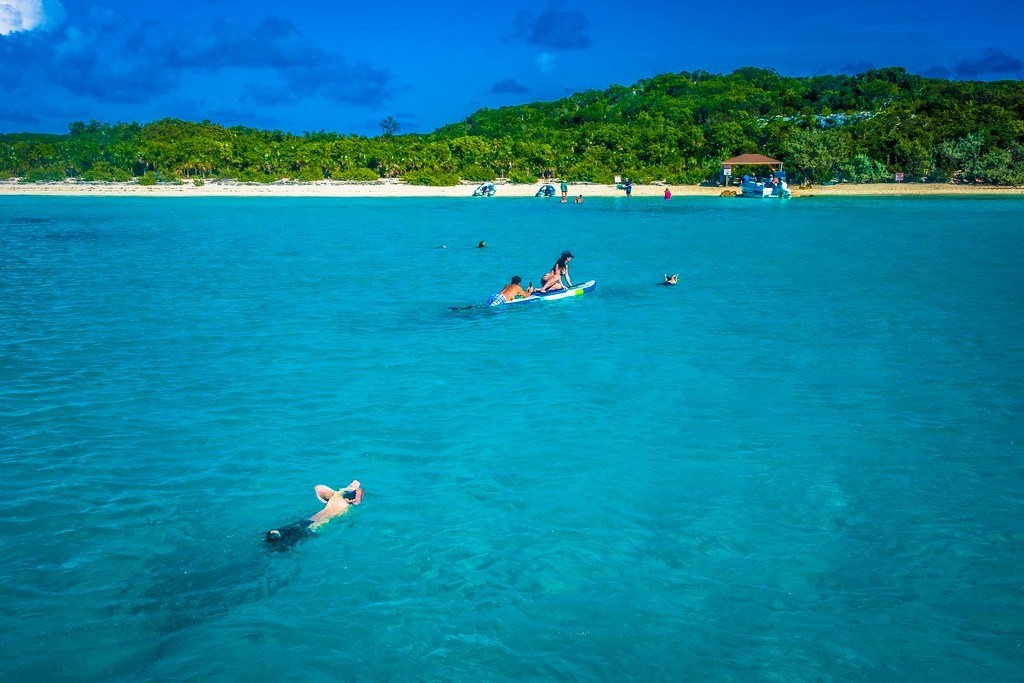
[504,279,596,306]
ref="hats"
[563,179,567,182]
[562,251,574,258]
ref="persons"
[488,276,533,306]
[665,188,671,200]
[561,196,568,203]
[578,195,583,203]
[267,480,364,545]
[624,177,632,197]
[534,251,574,293]
[561,179,568,196]
[574,196,578,204]
[664,274,677,285]
